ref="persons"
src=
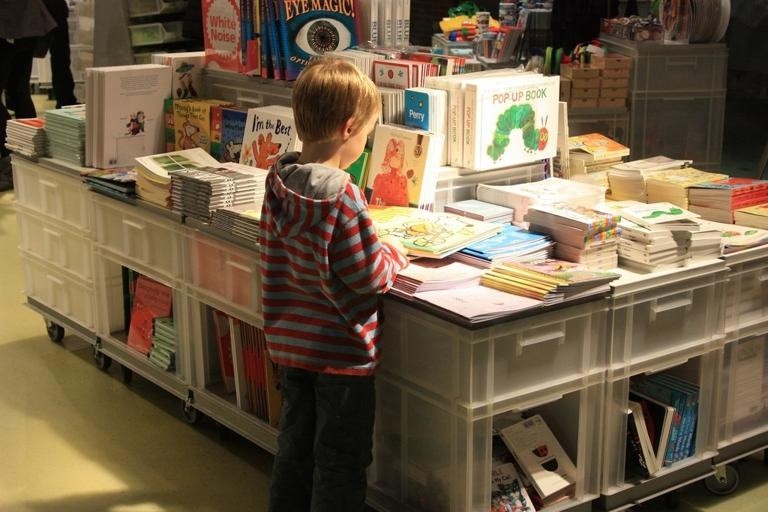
[260,62,409,512]
[1,0,74,118]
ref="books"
[4,50,303,243]
[213,310,281,426]
[202,1,410,81]
[326,46,768,323]
[491,415,576,512]
[625,372,700,478]
[127,269,176,369]
